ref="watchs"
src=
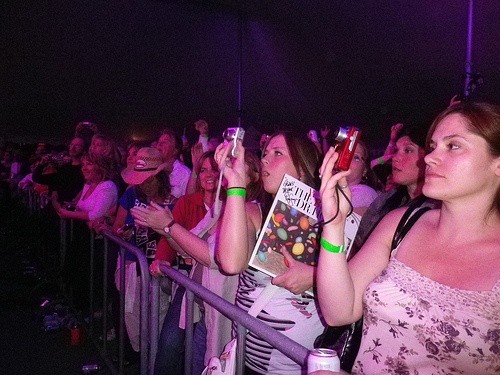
[163,219,176,233]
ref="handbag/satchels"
[205,337,237,375]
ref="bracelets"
[320,238,346,253]
[226,186,247,190]
[226,188,246,197]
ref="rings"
[318,175,322,179]
[144,218,147,222]
[338,184,348,189]
[216,158,221,162]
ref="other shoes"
[99,327,116,342]
[95,305,112,318]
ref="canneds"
[82,364,100,374]
[307,348,340,375]
[40,298,63,331]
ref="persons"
[0,116,441,375]
[316,90,500,375]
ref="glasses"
[352,153,367,164]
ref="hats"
[121,147,174,185]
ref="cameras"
[223,126,245,158]
[333,125,362,171]
